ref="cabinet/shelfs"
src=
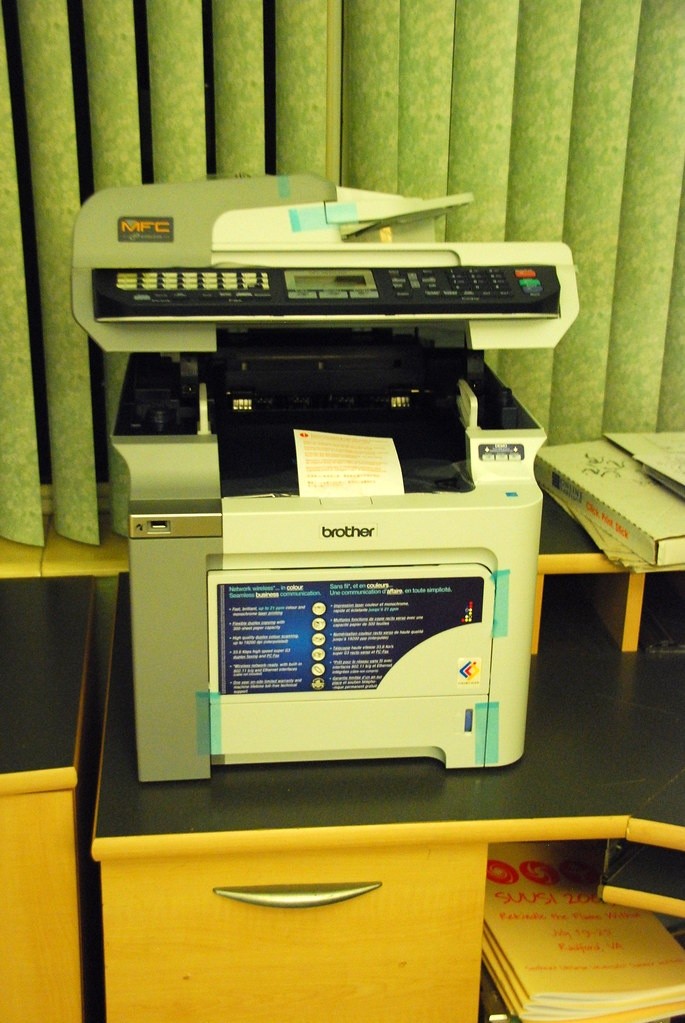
[0,575,96,1023]
[89,571,685,1023]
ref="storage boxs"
[534,439,685,567]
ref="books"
[534,440,685,568]
[482,840,684,1022]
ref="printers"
[68,174,580,781]
